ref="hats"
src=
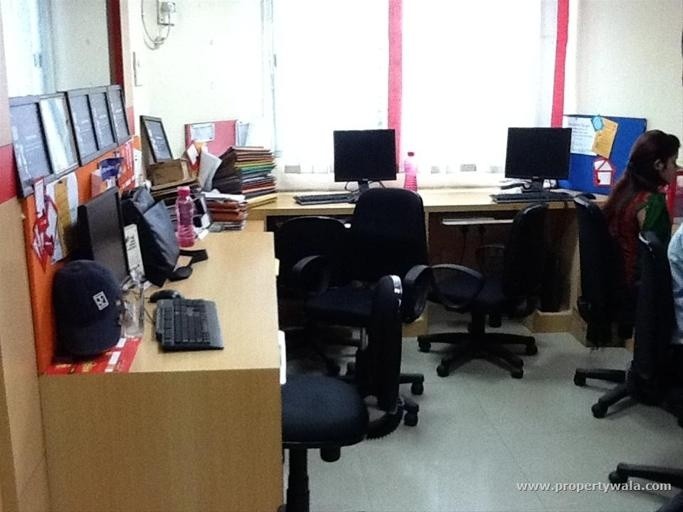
[53,260,127,356]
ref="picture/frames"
[141,115,173,162]
[184,140,199,165]
[8,85,131,197]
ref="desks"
[41,201,282,512]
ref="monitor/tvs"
[505,127,572,192]
[78,187,134,299]
[333,129,396,195]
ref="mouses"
[350,197,360,203]
[576,192,596,199]
[150,290,182,302]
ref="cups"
[123,290,145,341]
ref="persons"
[667,220,683,381]
[600,128,680,325]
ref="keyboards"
[489,192,574,203]
[293,194,351,204]
[156,299,224,351]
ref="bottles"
[403,151,417,193]
[173,187,194,247]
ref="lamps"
[141,0,176,50]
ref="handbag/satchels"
[120,186,209,288]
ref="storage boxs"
[148,158,190,185]
